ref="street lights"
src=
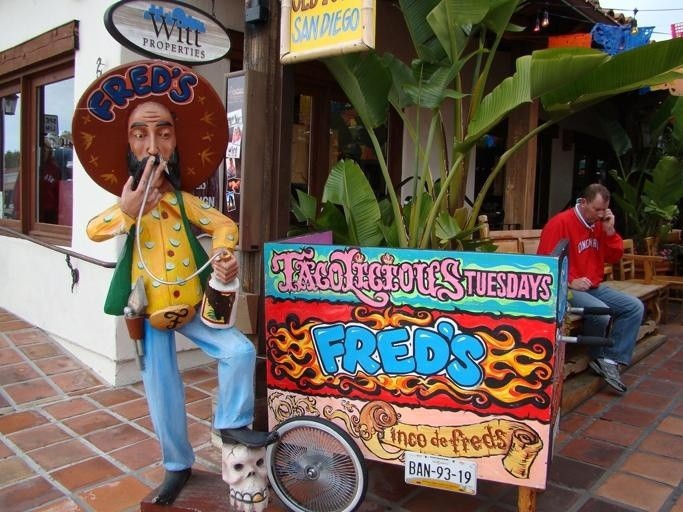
[2,93,20,116]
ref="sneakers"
[587,357,627,392]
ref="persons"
[69,58,281,511]
[10,139,61,224]
[531,184,645,395]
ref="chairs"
[619,236,683,301]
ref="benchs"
[475,225,669,423]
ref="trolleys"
[256,229,620,512]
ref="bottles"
[200,251,240,329]
